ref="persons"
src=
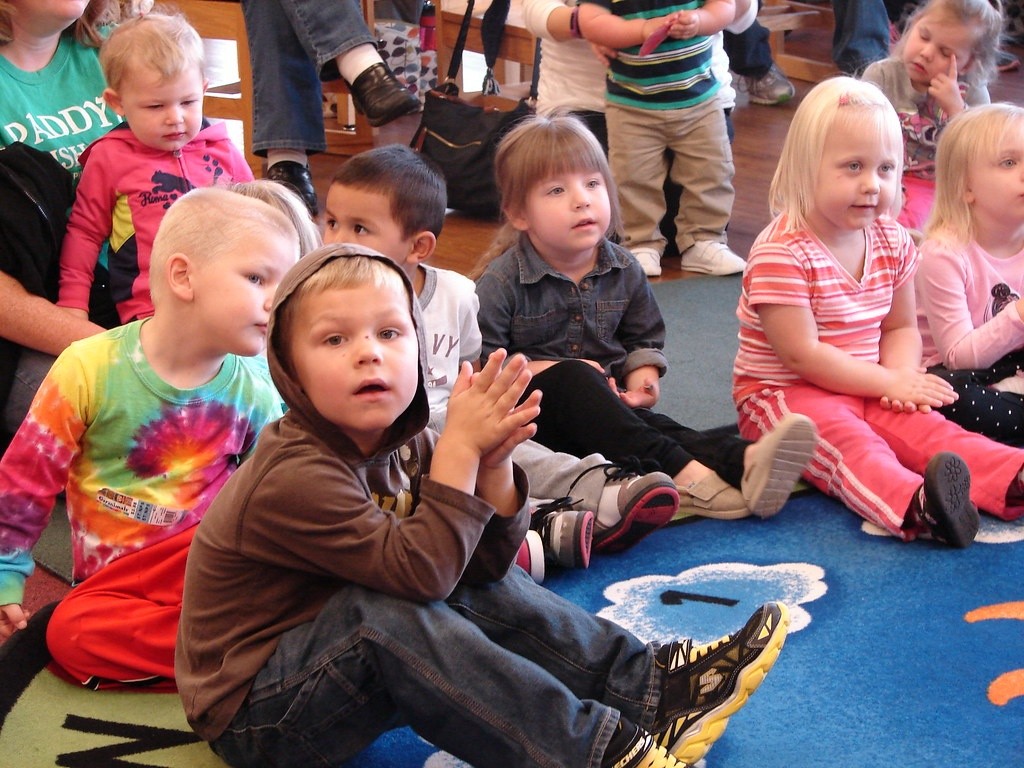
[233,180,545,583]
[323,141,679,568]
[0,186,548,690]
[243,0,423,219]
[57,11,254,324]
[470,110,817,519]
[525,0,1024,277]
[733,76,1024,546]
[0,0,126,495]
[917,99,1024,447]
[175,241,790,768]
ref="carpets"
[0,271,1024,768]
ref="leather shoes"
[266,156,320,217]
[345,53,421,130]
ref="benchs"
[431,0,843,116]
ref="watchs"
[570,7,583,40]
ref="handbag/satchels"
[408,75,544,223]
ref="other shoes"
[735,61,796,105]
[914,449,982,549]
[671,468,752,521]
[739,413,819,518]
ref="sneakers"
[627,247,663,277]
[599,717,697,768]
[586,456,682,555]
[679,238,747,276]
[515,526,548,584]
[644,599,791,765]
[530,494,594,571]
